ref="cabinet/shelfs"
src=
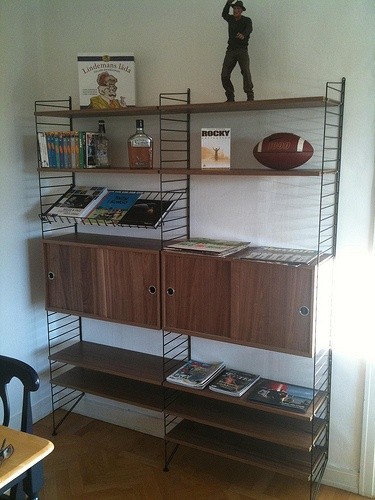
[35,76,346,500]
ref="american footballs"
[253,133,313,170]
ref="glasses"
[0,439,14,466]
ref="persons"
[222,0,254,102]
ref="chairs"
[0,355,40,500]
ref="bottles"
[94,119,113,169]
[127,118,153,169]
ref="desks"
[0,425,54,500]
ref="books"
[43,185,251,257]
[166,360,318,414]
[37,131,98,168]
